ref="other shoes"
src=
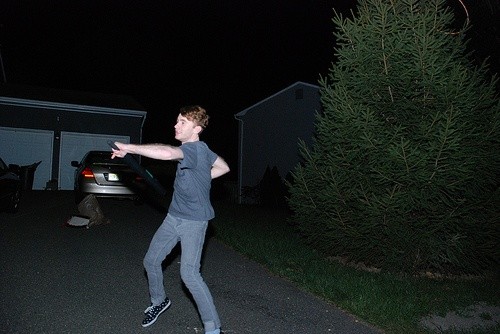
[141,296,171,328]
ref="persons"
[112,105,231,334]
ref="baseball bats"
[105,138,167,194]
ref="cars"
[72,149,131,207]
[0,158,22,212]
